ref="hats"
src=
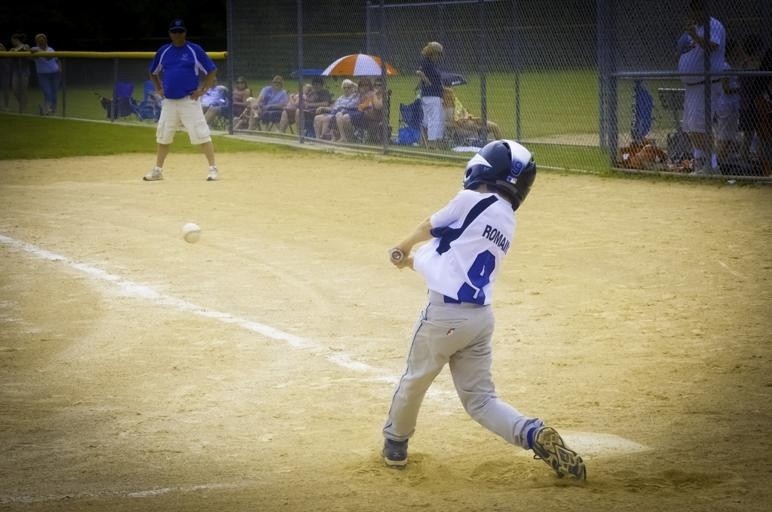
[170,19,186,30]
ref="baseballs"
[181,224,203,244]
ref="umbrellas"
[440,67,463,88]
[322,50,397,78]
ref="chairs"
[97,81,390,144]
[399,88,490,149]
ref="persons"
[143,19,217,180]
[417,42,446,146]
[716,39,742,174]
[202,77,388,143]
[32,35,63,117]
[379,142,590,482]
[739,35,762,171]
[9,32,30,111]
[676,2,740,175]
[447,85,502,143]
[754,50,772,176]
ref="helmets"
[463,139,536,211]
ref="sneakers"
[144,170,164,181]
[533,426,587,480]
[207,169,217,180]
[381,439,408,467]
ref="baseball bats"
[390,248,404,264]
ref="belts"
[686,78,721,86]
